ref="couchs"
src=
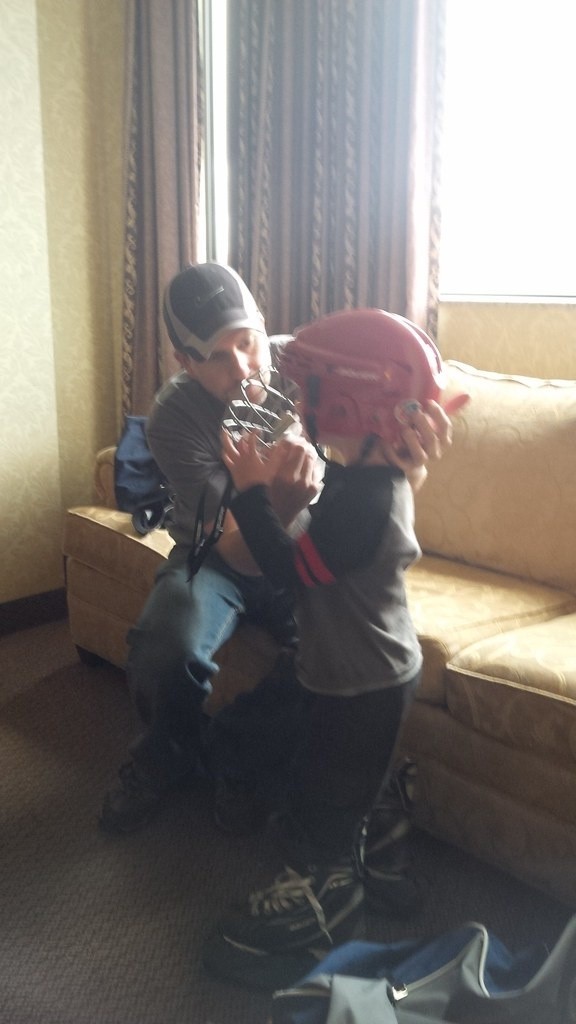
[69,358,576,907]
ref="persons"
[99,261,471,992]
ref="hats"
[163,261,265,363]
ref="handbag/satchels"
[267,917,576,1024]
[115,414,177,534]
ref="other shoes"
[222,867,364,952]
[99,750,200,830]
[210,737,256,833]
[357,807,409,854]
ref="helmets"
[280,308,443,439]
